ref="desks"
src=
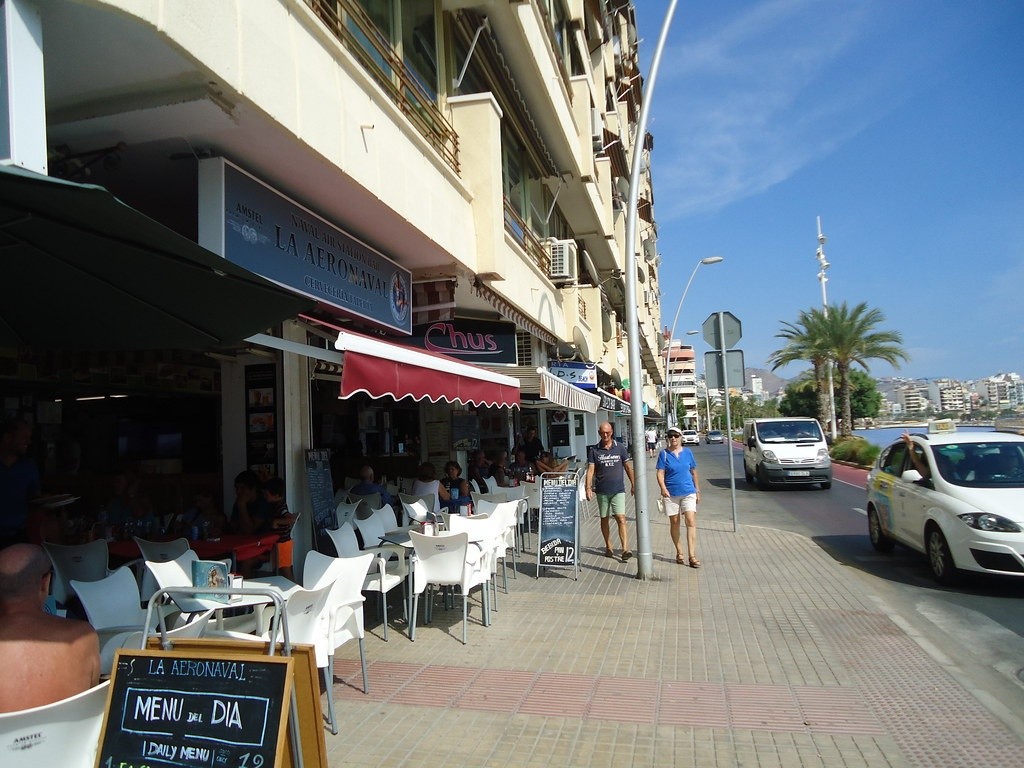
[108,533,279,577]
[166,576,305,624]
[469,496,532,549]
[568,461,578,470]
[412,514,468,523]
[0,455,590,768]
[377,531,488,627]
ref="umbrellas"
[0,160,315,353]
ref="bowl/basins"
[256,424,268,431]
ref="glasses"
[668,434,679,438]
[42,565,54,579]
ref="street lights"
[815,215,837,442]
[670,330,699,414]
[674,359,698,421]
[666,256,723,429]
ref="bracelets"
[586,487,592,490]
[238,502,245,504]
[697,491,700,492]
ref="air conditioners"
[644,291,649,304]
[551,243,579,285]
[516,330,548,368]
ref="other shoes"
[689,556,701,568]
[676,553,684,563]
[621,551,632,560]
[605,549,613,556]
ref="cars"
[866,418,1024,582]
[705,430,724,444]
[680,429,701,446]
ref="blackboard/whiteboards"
[305,447,339,557]
[93,648,295,768]
[451,409,480,452]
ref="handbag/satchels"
[657,499,665,513]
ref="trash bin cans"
[586,445,597,464]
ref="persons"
[999,446,1024,470]
[487,447,507,485]
[412,462,473,527]
[585,422,635,560]
[250,391,265,407]
[655,427,702,569]
[208,569,217,586]
[351,464,399,524]
[511,425,544,463]
[796,424,810,437]
[467,449,488,492]
[0,417,158,714]
[183,470,297,568]
[899,428,961,484]
[646,427,670,458]
[510,450,536,482]
[537,452,568,478]
[393,431,420,456]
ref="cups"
[451,488,459,500]
[234,575,243,590]
[459,506,468,516]
[254,391,262,401]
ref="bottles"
[445,484,450,493]
[504,466,535,487]
[193,524,199,540]
[203,521,209,540]
[277,536,293,569]
[468,501,476,515]
[64,513,87,533]
[98,504,110,525]
[414,436,420,452]
[123,520,153,539]
[553,452,560,466]
[510,448,516,464]
[404,434,410,452]
[259,418,265,426]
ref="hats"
[668,427,683,436]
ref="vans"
[743,417,833,489]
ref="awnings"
[486,365,603,414]
[594,388,632,416]
[245,314,521,409]
